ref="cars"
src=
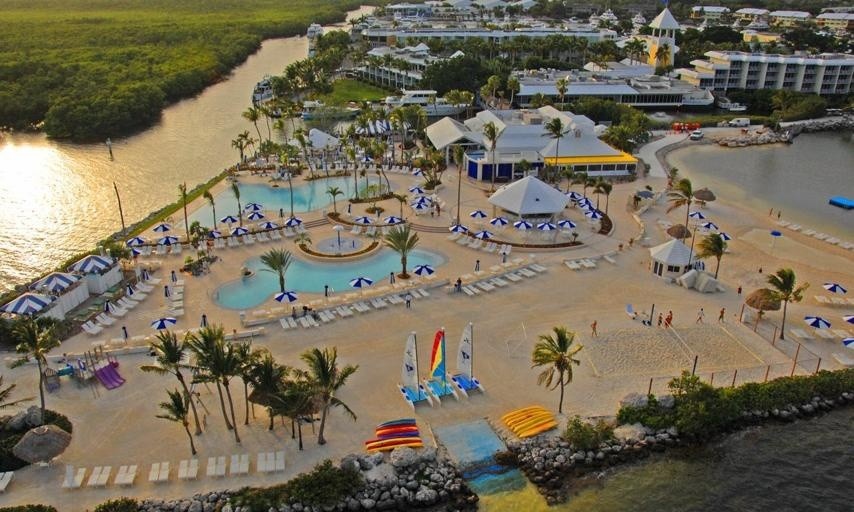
[690,130,704,140]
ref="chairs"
[84,276,164,336]
[446,232,510,255]
[165,278,184,318]
[0,452,285,494]
[308,165,426,177]
[349,225,408,240]
[460,255,546,296]
[130,223,309,258]
[95,326,206,347]
[790,328,854,366]
[255,281,428,332]
[565,258,595,271]
[776,220,854,249]
[430,193,447,210]
[814,295,854,305]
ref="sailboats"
[397,322,486,413]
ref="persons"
[62,353,69,367]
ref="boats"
[252,79,275,101]
[384,90,470,116]
[306,22,324,38]
[301,99,361,119]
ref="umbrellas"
[0,291,52,316]
[803,314,833,334]
[30,270,81,292]
[62,254,111,274]
[820,283,849,301]
[843,339,854,350]
[768,229,781,248]
[843,314,853,324]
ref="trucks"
[728,117,750,127]
